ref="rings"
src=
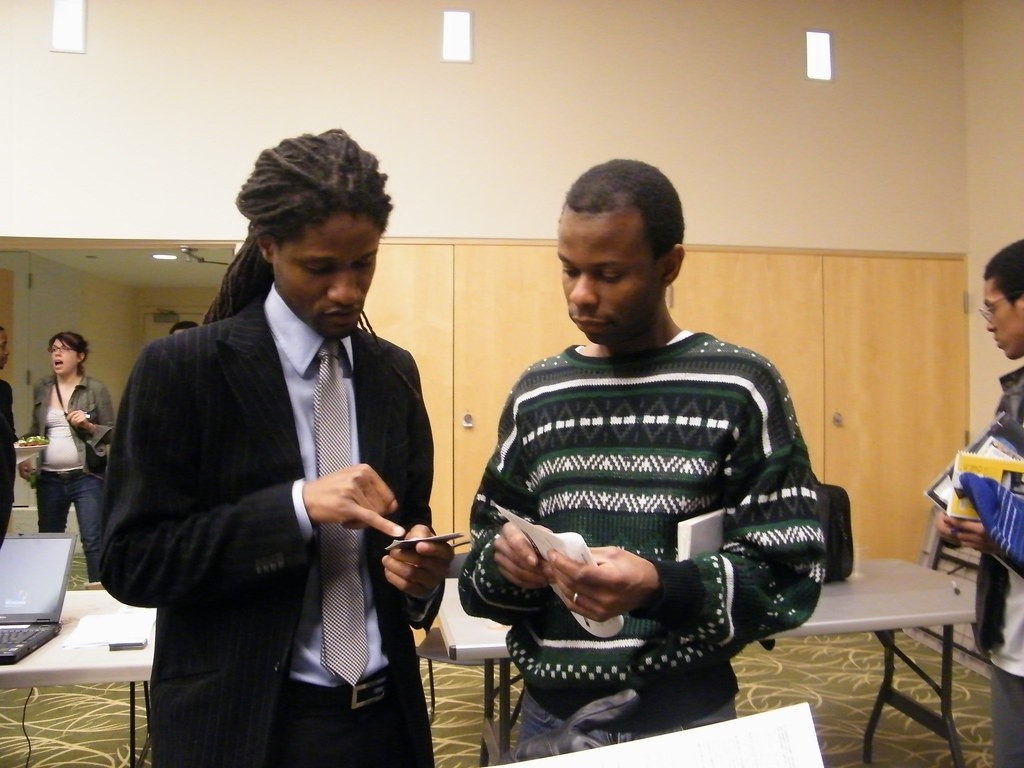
[572,592,579,604]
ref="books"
[922,410,1024,568]
[672,505,726,565]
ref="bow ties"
[315,339,371,689]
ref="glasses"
[980,290,1021,321]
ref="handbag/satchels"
[802,471,854,587]
[83,440,109,480]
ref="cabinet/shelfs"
[352,235,642,550]
[655,242,971,562]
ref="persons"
[0,327,22,547]
[18,331,119,586]
[937,238,1024,766]
[90,125,455,766]
[167,320,199,336]
[457,157,834,758]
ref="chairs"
[415,552,524,731]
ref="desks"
[0,590,158,768]
[438,558,980,768]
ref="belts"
[41,466,85,480]
[283,678,391,716]
